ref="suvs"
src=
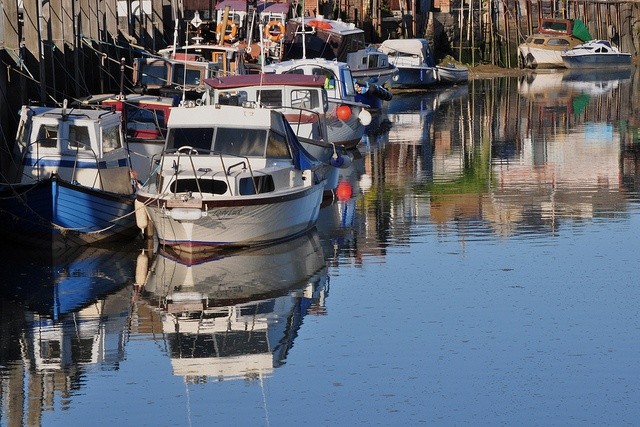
[517,72,575,106]
[518,32,583,70]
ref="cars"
[517,72,582,103]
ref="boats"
[157,45,245,78]
[133,58,219,107]
[132,226,327,384]
[267,15,399,89]
[560,39,631,70]
[0,234,145,374]
[0,94,174,233]
[259,58,371,149]
[377,39,468,88]
[204,75,328,143]
[563,78,620,97]
[216,0,255,54]
[134,105,329,247]
[257,4,295,58]
[386,85,467,145]
